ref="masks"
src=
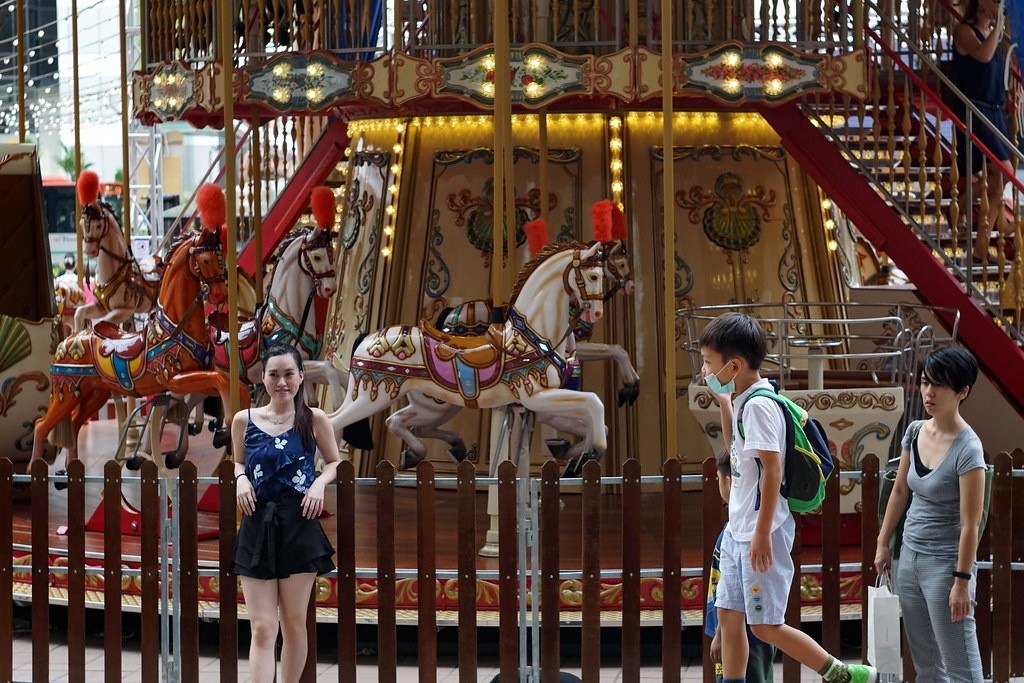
[703,360,737,394]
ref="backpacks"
[738,386,835,513]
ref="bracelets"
[234,474,246,481]
[951,570,971,580]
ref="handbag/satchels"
[867,562,901,674]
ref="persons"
[869,347,987,683]
[949,1,1021,268]
[695,311,876,683]
[228,345,341,683]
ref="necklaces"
[265,405,297,425]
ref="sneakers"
[845,664,877,683]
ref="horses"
[127,224,337,469]
[314,239,643,478]
[74,199,161,333]
[26,225,250,490]
